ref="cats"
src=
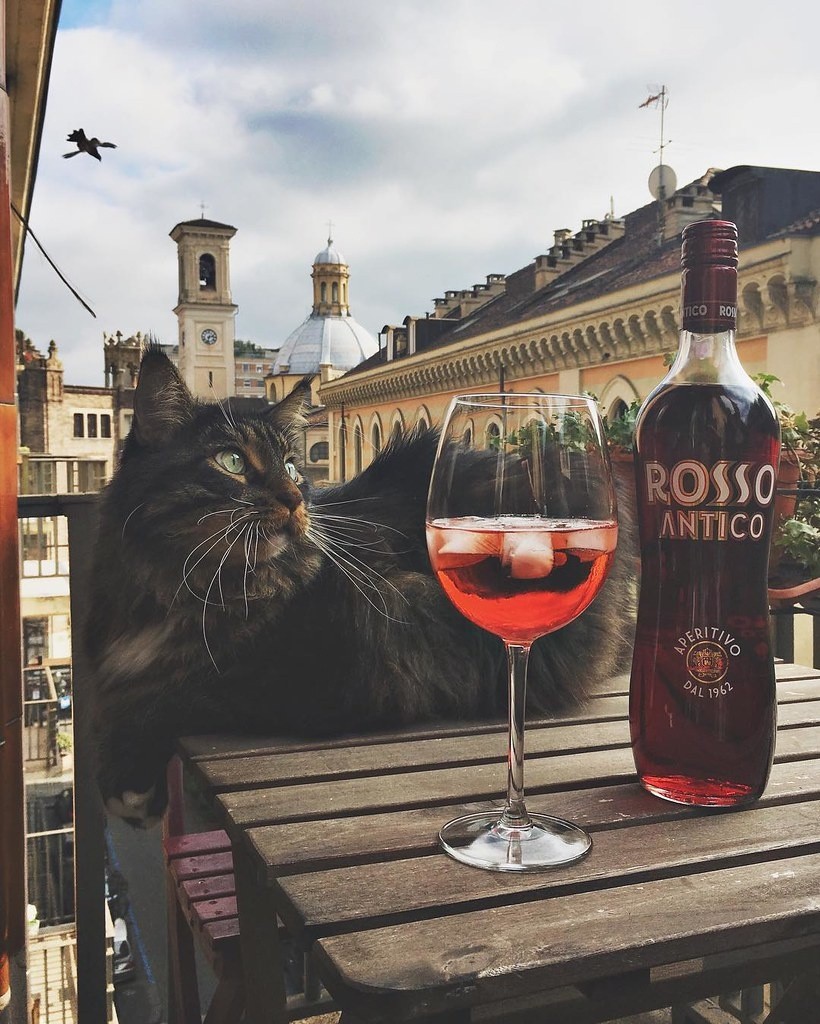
[79,338,636,828]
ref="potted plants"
[598,356,813,585]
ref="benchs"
[160,754,763,1024]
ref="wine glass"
[427,395,617,877]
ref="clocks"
[201,329,217,346]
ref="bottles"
[630,220,781,807]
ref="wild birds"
[61,128,118,162]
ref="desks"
[171,652,820,1024]
